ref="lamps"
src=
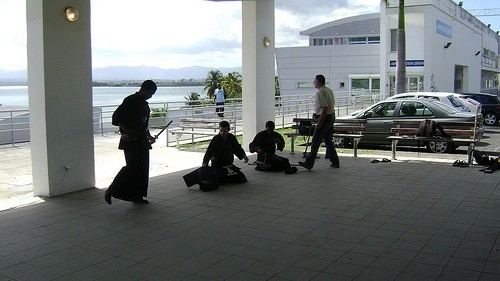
[444,42,452,48]
[475,51,480,55]
[459,2,463,6]
[496,31,499,34]
[488,24,491,27]
[64,5,79,22]
[264,36,271,47]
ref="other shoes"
[298,161,311,171]
[330,164,339,168]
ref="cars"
[457,92,500,127]
[350,91,483,118]
[329,96,485,154]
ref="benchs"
[169,117,235,150]
[283,118,367,159]
[387,120,480,165]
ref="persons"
[202,121,249,185]
[298,75,339,170]
[105,80,157,205]
[213,83,226,117]
[249,121,291,172]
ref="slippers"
[453,160,460,166]
[459,160,469,166]
[371,160,380,163]
[381,158,391,162]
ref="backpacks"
[417,120,436,137]
[473,150,489,165]
